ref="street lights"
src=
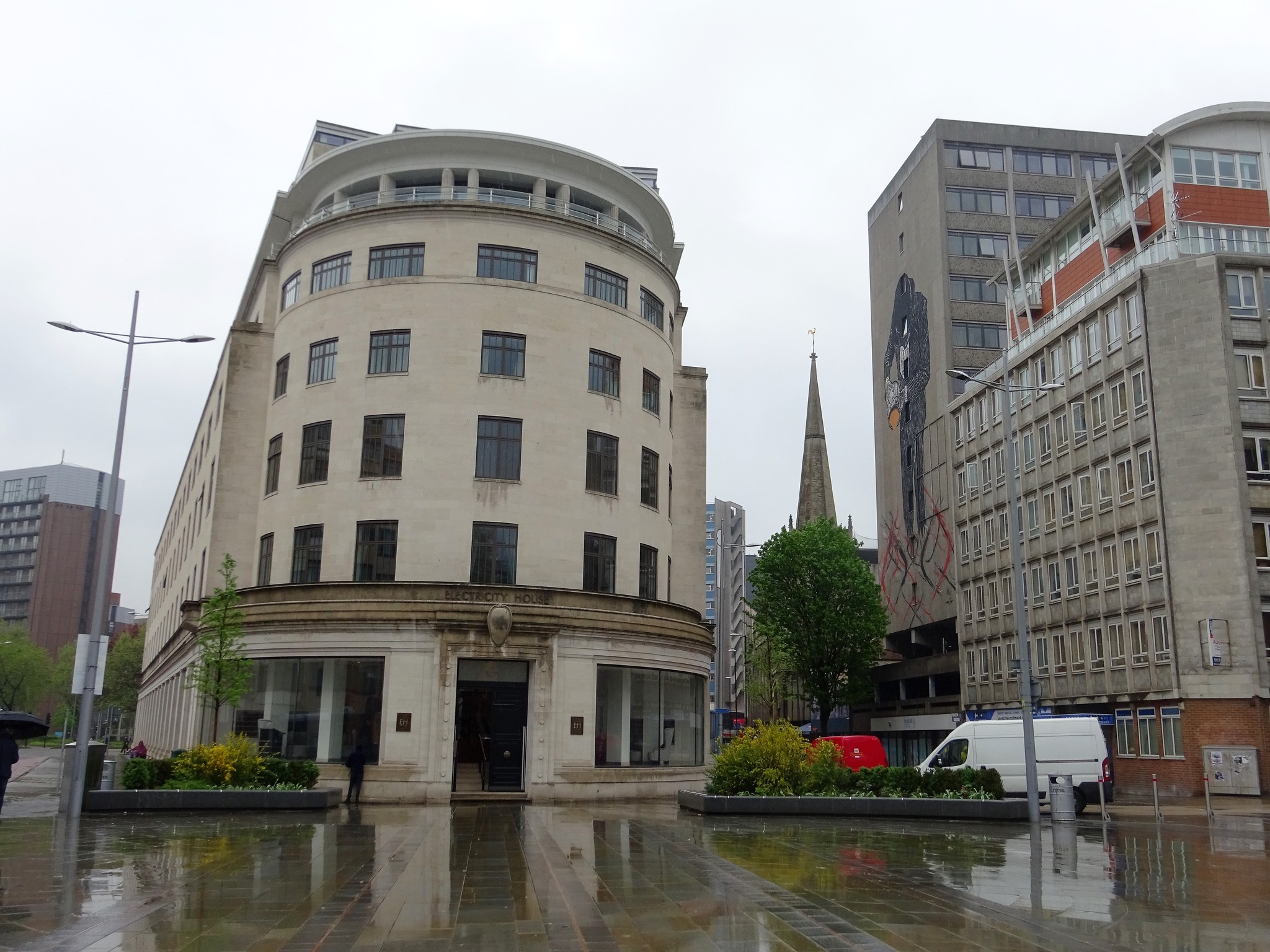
[730,633,748,727]
[47,289,217,819]
[729,649,737,723]
[717,518,763,746]
[944,346,1066,823]
[725,676,731,738]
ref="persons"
[961,746,968,763]
[74,736,77,742]
[343,742,365,803]
[129,739,147,759]
[102,738,111,761]
[0,723,19,815]
[90,736,96,741]
[120,736,130,753]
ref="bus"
[722,710,747,743]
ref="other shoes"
[355,798,359,802]
[342,798,350,803]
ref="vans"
[805,735,889,773]
[914,716,1113,816]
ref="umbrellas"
[0,711,50,740]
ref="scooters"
[127,750,136,759]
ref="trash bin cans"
[101,760,117,790]
[1048,773,1076,820]
